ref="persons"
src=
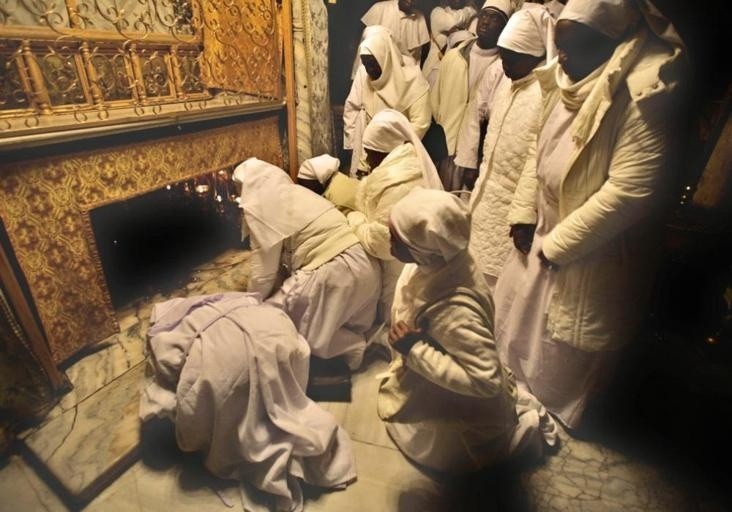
[371,188,560,483]
[341,0,701,445]
[298,152,364,217]
[231,156,398,402]
[138,287,361,508]
